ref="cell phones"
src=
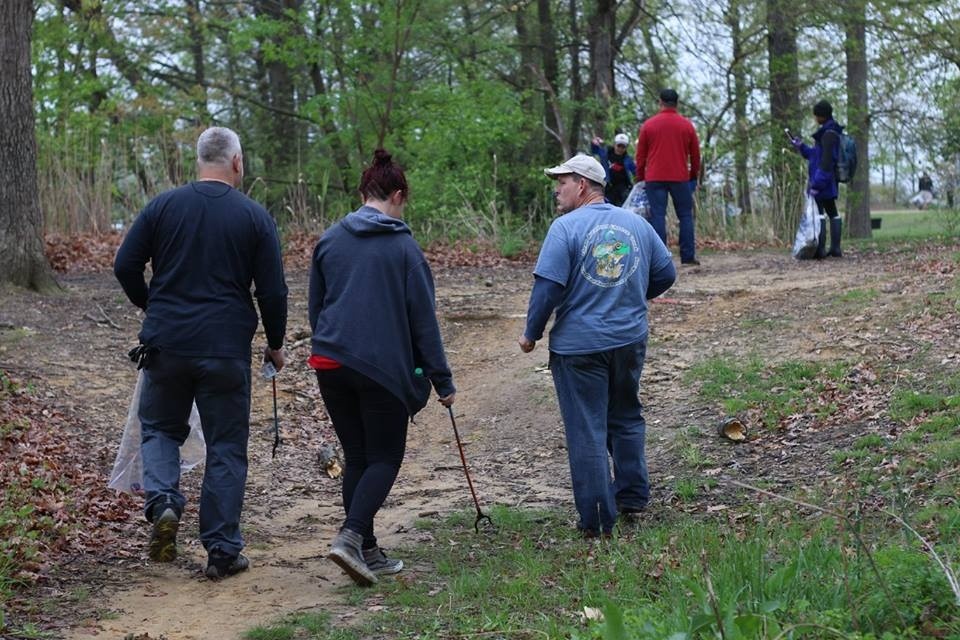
[785,128,796,143]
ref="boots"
[813,217,828,261]
[825,217,843,258]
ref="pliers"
[272,372,280,460]
[449,407,495,534]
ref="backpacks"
[821,129,857,183]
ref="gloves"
[809,189,819,196]
[791,135,802,147]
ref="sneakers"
[206,547,249,579]
[328,527,379,587]
[361,546,403,576]
[149,493,183,564]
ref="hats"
[614,133,630,147]
[543,156,607,187]
[813,99,833,118]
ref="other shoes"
[622,508,643,518]
[681,258,701,267]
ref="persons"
[918,172,933,209]
[310,149,455,585]
[518,154,677,538]
[591,134,636,207]
[115,128,288,579]
[636,89,701,266]
[792,99,845,258]
